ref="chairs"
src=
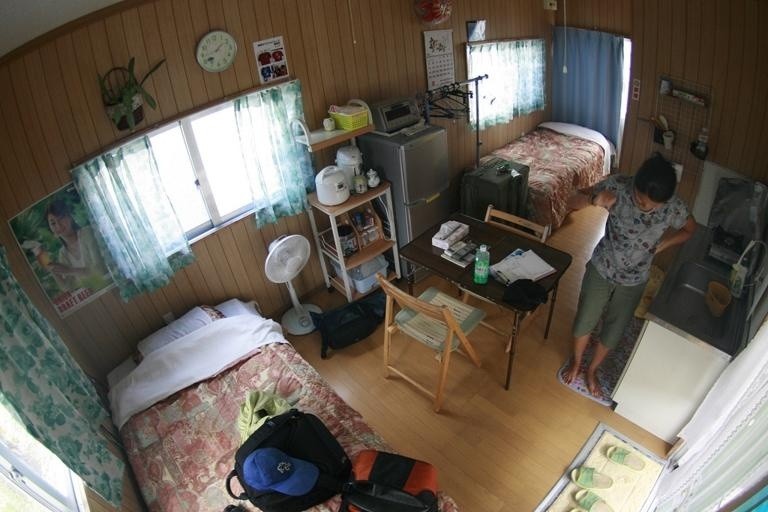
[484,204,549,243]
[375,273,486,411]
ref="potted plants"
[100,68,145,132]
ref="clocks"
[195,31,237,73]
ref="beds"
[106,299,459,511]
[480,122,615,229]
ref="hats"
[243,449,318,496]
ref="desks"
[400,212,573,391]
[613,222,755,446]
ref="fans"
[264,233,322,335]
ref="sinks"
[667,260,738,339]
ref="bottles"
[474,245,489,284]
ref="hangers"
[419,82,473,120]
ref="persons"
[30,200,108,293]
[561,155,697,398]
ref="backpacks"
[225,408,350,512]
[344,450,437,512]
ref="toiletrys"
[335,210,383,259]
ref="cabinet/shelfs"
[289,99,401,302]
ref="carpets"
[634,263,663,319]
[530,419,665,512]
[558,316,644,406]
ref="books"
[441,235,495,268]
[489,249,557,288]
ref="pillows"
[133,305,227,366]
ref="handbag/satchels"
[321,301,377,359]
